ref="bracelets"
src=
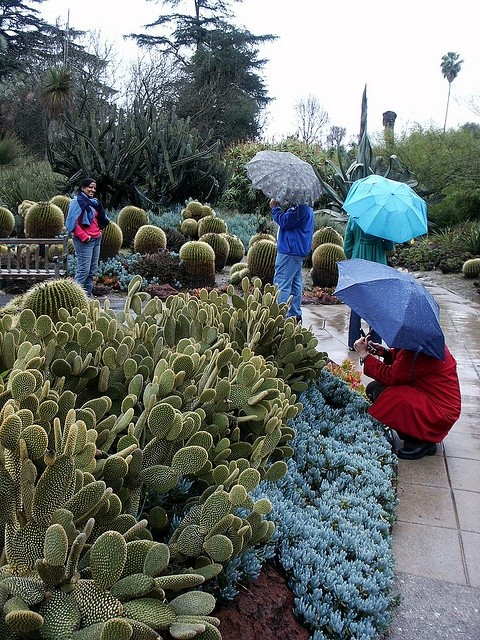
[87,235,91,242]
[360,354,371,366]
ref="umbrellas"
[244,150,323,207]
[340,172,428,244]
[332,257,445,360]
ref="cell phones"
[360,328,378,354]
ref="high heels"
[398,442,437,459]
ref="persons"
[65,178,110,296]
[267,197,315,324]
[353,335,461,460]
[343,215,395,350]
